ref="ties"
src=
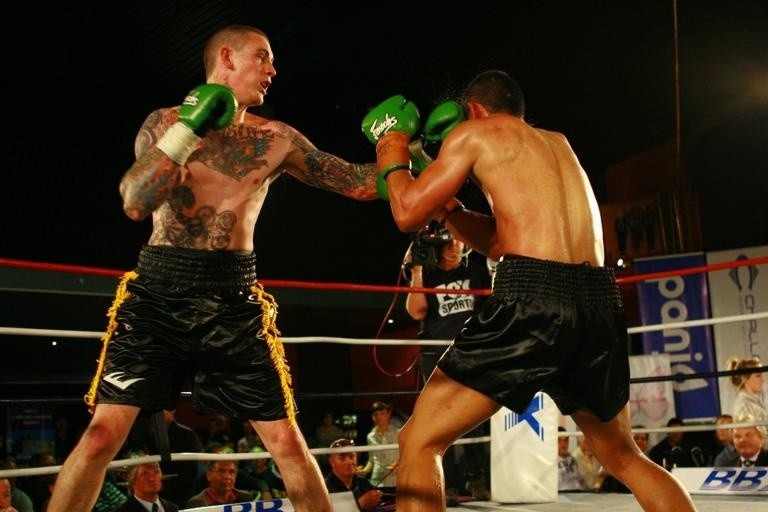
[150,504,157,512]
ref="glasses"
[331,439,356,448]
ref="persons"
[550,414,768,495]
[726,354,766,452]
[0,398,491,512]
[358,69,698,512]
[46,25,469,512]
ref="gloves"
[156,81,240,169]
[409,102,470,175]
[372,152,463,225]
[360,93,423,178]
